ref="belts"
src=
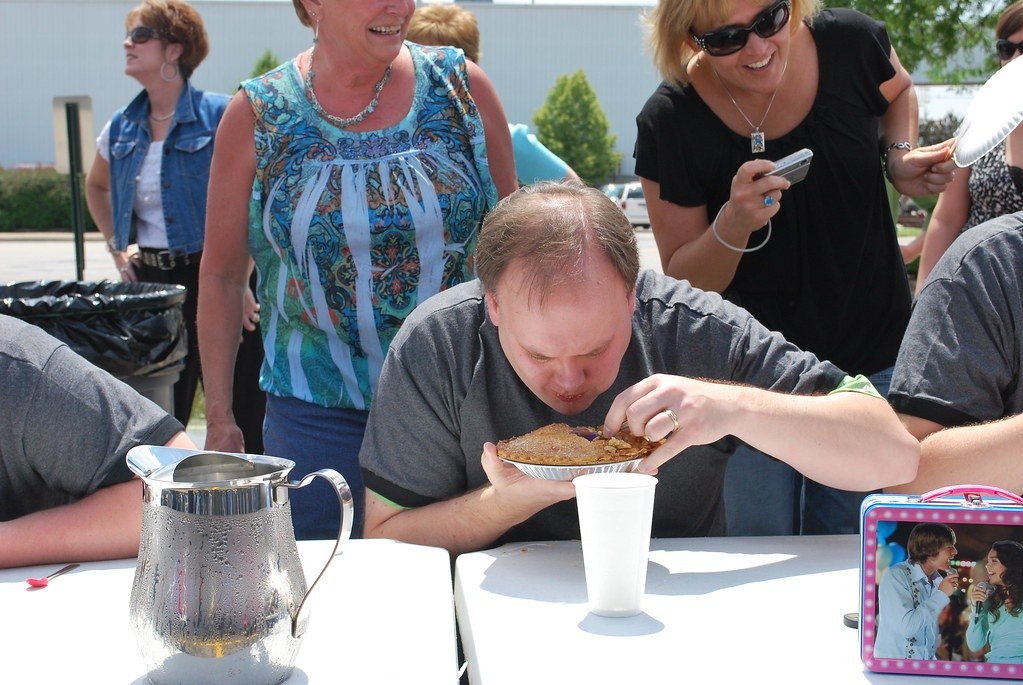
[138,252,191,271]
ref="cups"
[572,473,659,617]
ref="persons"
[84,0,264,454]
[402,4,583,184]
[359,179,921,556]
[884,209,1023,500]
[966,540,1023,665]
[631,0,958,539]
[198,1,519,543]
[874,525,959,661]
[0,314,199,570]
[898,3,1023,295]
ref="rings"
[956,583,958,587]
[251,312,257,321]
[763,194,774,206]
[663,407,680,433]
[121,267,128,272]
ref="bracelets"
[712,202,772,253]
[883,140,912,183]
[107,235,119,256]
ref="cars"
[599,183,622,204]
[611,182,650,229]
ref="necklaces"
[147,111,176,122]
[713,59,787,154]
[306,46,393,126]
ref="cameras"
[762,147,813,185]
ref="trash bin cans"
[0,282,186,421]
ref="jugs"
[125,445,354,685]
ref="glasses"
[689,0,791,55]
[996,40,1023,60]
[125,27,162,43]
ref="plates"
[953,54,1023,170]
[495,456,643,480]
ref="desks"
[454,533,1022,685]
[0,537,460,685]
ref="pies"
[497,424,665,466]
